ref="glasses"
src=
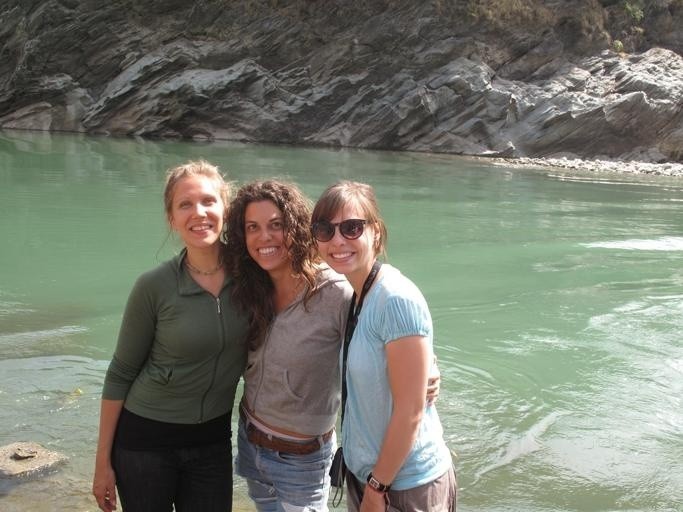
[308,219,377,242]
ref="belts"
[238,403,335,456]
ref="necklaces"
[184,256,224,275]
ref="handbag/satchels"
[329,447,347,488]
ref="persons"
[95,161,251,512]
[226,179,440,512]
[308,181,457,512]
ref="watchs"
[367,472,391,492]
